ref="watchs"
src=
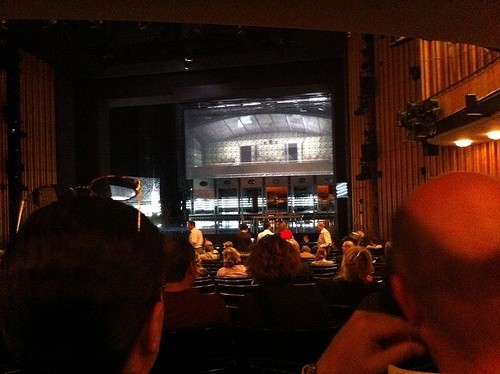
[301,364,317,374]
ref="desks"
[252,215,304,234]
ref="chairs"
[149,246,387,374]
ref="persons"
[162,234,229,324]
[0,195,166,374]
[280,220,391,285]
[187,220,273,278]
[233,235,328,332]
[301,172,500,374]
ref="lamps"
[356,144,382,178]
[465,94,488,117]
[396,99,440,134]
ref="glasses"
[17,175,141,232]
